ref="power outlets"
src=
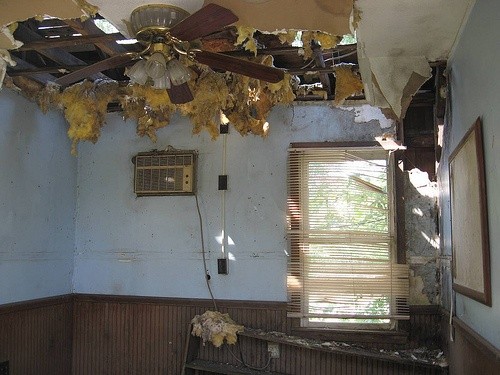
[268,342,280,358]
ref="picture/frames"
[448,116,492,307]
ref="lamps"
[126,44,191,90]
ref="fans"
[53,3,284,86]
